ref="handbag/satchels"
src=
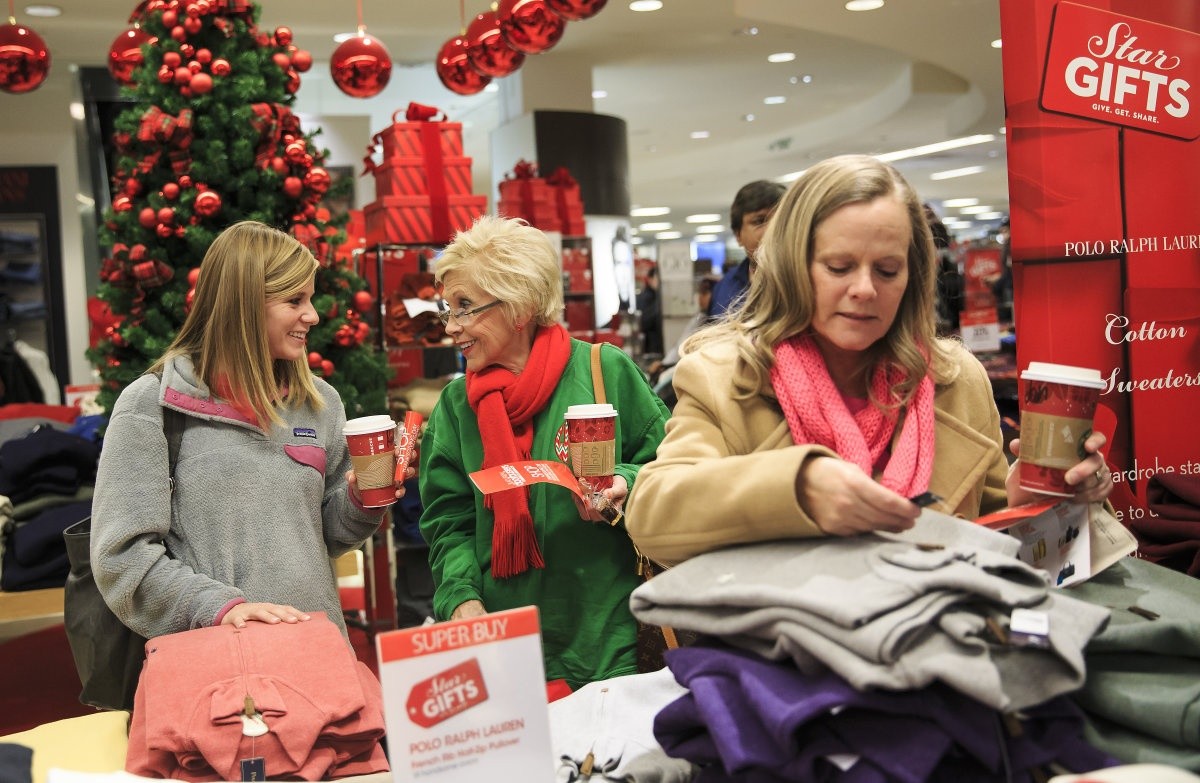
[637,560,665,675]
[59,362,186,717]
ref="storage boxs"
[381,122,462,161]
[496,175,586,235]
[365,194,486,246]
[372,156,474,197]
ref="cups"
[344,415,398,512]
[561,403,618,495]
[1016,358,1109,503]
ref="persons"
[91,220,416,635]
[638,266,666,386]
[991,217,1012,332]
[622,154,1114,568]
[417,217,672,694]
[920,200,964,338]
[706,179,788,326]
[0,291,62,408]
[645,274,721,413]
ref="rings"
[1095,471,1103,484]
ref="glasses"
[437,295,521,325]
